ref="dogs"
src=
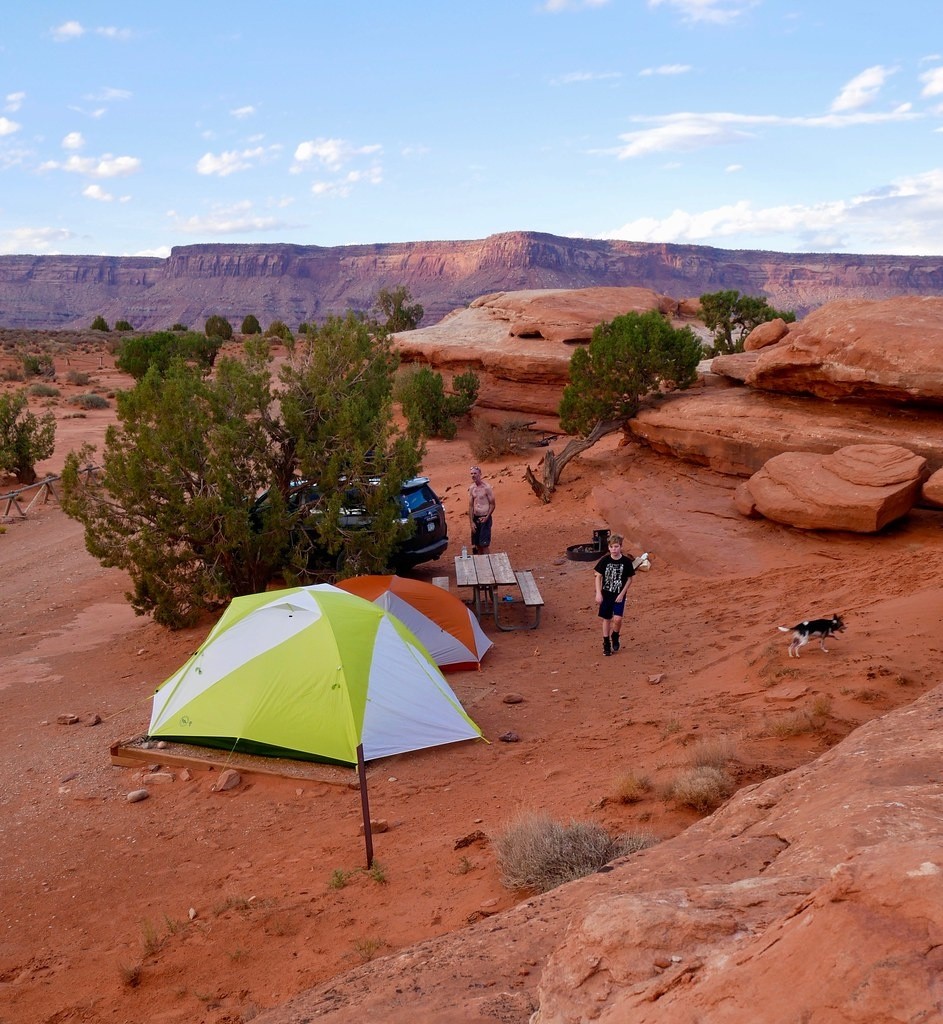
[778,613,846,658]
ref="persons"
[467,466,495,589]
[594,534,635,655]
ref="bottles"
[462,547,467,560]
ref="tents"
[145,584,491,782]
[333,575,493,672]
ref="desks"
[454,552,519,632]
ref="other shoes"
[603,642,612,656]
[611,634,620,651]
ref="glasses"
[470,466,479,470]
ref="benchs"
[514,572,544,629]
[431,576,449,591]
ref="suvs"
[233,450,450,577]
